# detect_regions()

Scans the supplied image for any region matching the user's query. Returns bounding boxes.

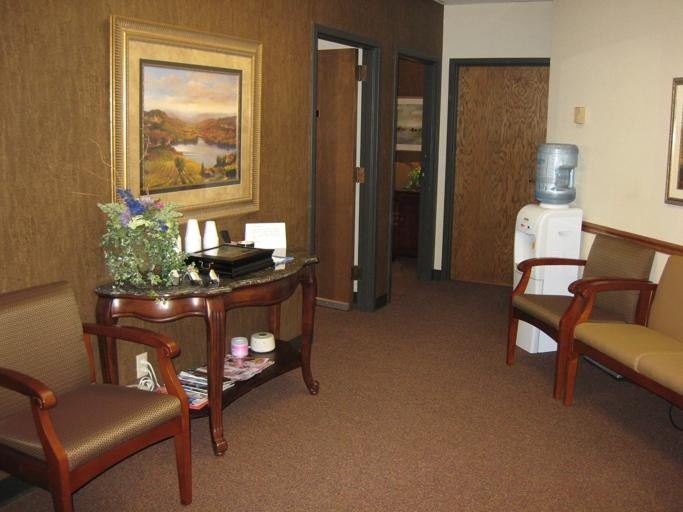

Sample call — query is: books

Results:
[151,352,275,411]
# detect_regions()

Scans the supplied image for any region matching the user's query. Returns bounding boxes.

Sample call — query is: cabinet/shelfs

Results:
[394,190,418,258]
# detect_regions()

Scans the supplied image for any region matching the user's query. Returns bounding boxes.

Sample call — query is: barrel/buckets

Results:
[533,143,579,204]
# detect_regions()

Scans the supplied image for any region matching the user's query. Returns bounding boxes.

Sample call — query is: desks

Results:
[91,253,321,453]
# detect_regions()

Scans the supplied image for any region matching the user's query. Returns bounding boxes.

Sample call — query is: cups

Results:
[183,218,221,251]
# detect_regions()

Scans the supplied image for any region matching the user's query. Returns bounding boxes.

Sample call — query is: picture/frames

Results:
[396,96,422,151]
[109,14,263,229]
[664,78,682,204]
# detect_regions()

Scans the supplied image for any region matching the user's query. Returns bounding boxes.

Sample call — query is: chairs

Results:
[506,220,683,412]
[2,280,191,512]
